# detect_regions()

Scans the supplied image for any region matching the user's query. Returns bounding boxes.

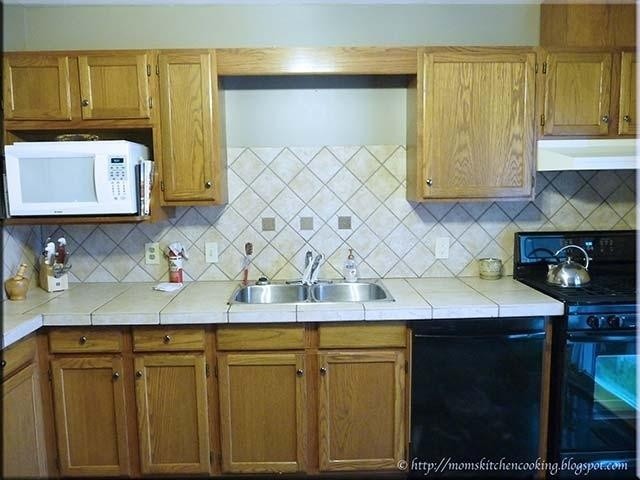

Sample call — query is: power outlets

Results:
[435,236,449,261]
[144,243,160,265]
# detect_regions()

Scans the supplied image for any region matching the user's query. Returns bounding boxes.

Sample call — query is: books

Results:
[145,159,154,217]
[133,162,141,217]
[140,160,145,217]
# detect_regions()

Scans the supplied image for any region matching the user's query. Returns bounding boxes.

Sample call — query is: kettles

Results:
[546,245,594,289]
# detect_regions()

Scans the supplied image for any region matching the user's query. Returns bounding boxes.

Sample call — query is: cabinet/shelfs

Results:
[406,54,534,204]
[132,329,214,479]
[48,329,126,477]
[0,333,49,479]
[160,50,229,208]
[314,324,405,472]
[1,52,153,121]
[215,327,310,476]
[536,4,638,139]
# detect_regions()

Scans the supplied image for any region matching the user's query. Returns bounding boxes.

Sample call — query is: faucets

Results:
[304,251,322,282]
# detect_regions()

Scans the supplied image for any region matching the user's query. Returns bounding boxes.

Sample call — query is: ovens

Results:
[551,330,640,478]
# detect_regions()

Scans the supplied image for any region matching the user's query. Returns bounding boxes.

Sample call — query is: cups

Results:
[478,257,501,281]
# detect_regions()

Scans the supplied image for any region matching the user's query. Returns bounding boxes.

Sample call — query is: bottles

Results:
[168,243,183,283]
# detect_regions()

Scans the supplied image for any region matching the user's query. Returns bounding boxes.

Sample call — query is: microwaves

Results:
[4,139,149,218]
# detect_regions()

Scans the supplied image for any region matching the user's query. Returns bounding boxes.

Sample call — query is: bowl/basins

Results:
[5,277,29,301]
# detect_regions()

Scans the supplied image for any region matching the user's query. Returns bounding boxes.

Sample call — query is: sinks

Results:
[310,278,396,303]
[227,280,310,305]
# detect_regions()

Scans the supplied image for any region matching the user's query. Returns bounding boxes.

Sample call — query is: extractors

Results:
[537,140,639,173]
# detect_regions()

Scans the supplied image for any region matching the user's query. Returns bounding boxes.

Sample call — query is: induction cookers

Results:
[519,273,637,331]
